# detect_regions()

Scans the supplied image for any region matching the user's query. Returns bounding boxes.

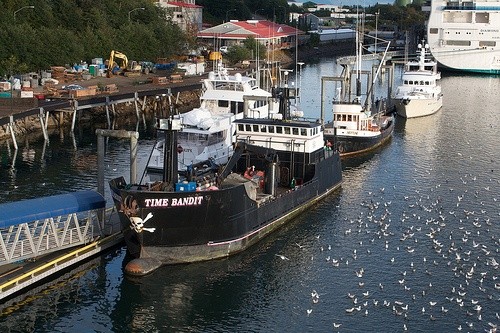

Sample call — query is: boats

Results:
[108,85,341,277]
[391,40,444,119]
[423,0,500,75]
[143,21,309,184]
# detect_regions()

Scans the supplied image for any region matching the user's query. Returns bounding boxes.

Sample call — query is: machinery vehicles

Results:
[105,49,143,80]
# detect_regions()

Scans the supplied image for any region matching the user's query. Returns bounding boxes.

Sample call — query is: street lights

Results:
[128,8,145,23]
[226,9,236,21]
[13,6,34,22]
[279,6,286,23]
[255,9,264,20]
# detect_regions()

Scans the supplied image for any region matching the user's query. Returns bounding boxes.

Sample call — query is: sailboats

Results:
[319,2,408,160]
